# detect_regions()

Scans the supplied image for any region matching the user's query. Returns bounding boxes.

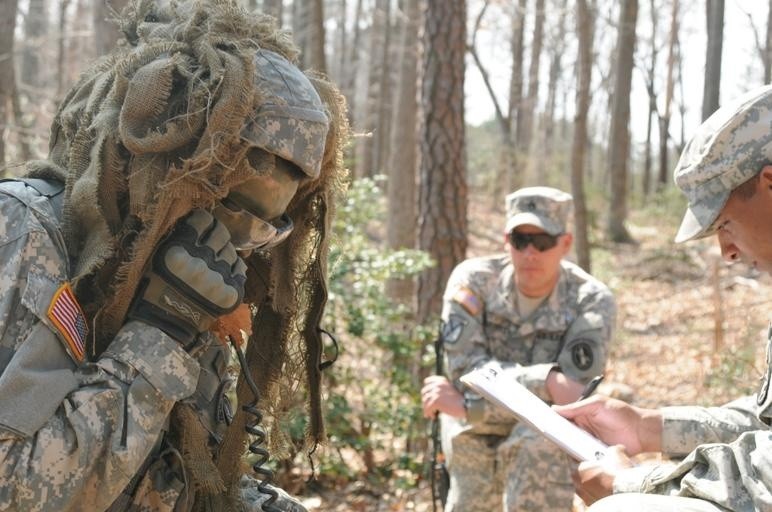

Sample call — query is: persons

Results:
[542,84,772,511]
[418,184,615,512]
[0,0,376,511]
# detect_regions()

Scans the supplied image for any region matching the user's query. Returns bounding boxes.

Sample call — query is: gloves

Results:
[131,209,245,348]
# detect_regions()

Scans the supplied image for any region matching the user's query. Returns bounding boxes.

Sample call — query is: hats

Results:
[673,84,772,245]
[503,186,573,236]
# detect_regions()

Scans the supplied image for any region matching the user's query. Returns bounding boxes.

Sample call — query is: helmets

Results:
[233,45,327,181]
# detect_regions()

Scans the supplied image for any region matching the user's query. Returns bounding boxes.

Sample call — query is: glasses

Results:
[507,231,562,252]
[205,198,295,252]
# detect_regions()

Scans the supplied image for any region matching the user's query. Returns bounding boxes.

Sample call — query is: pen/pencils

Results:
[579,375,604,400]
